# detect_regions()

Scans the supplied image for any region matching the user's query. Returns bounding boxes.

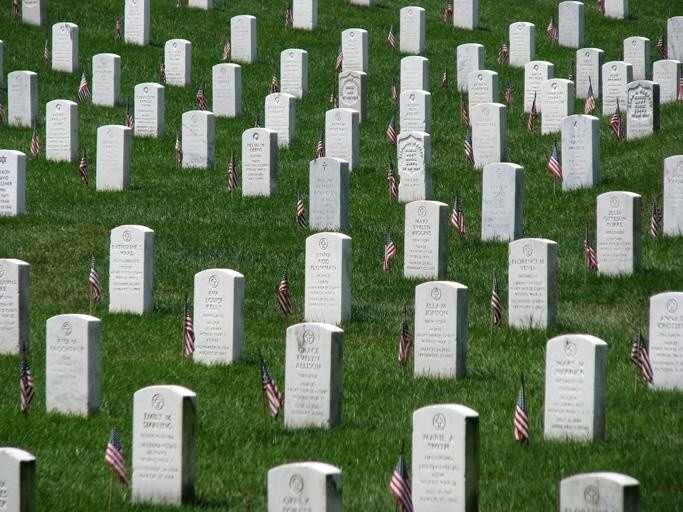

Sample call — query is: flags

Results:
[104,425,130,486]
[87,254,102,302]
[18,352,36,414]
[182,304,197,358]
[395,317,414,365]
[490,277,503,328]
[387,453,414,511]
[258,358,283,420]
[275,268,294,317]
[13,1,683,279]
[628,324,655,388]
[512,383,531,444]
